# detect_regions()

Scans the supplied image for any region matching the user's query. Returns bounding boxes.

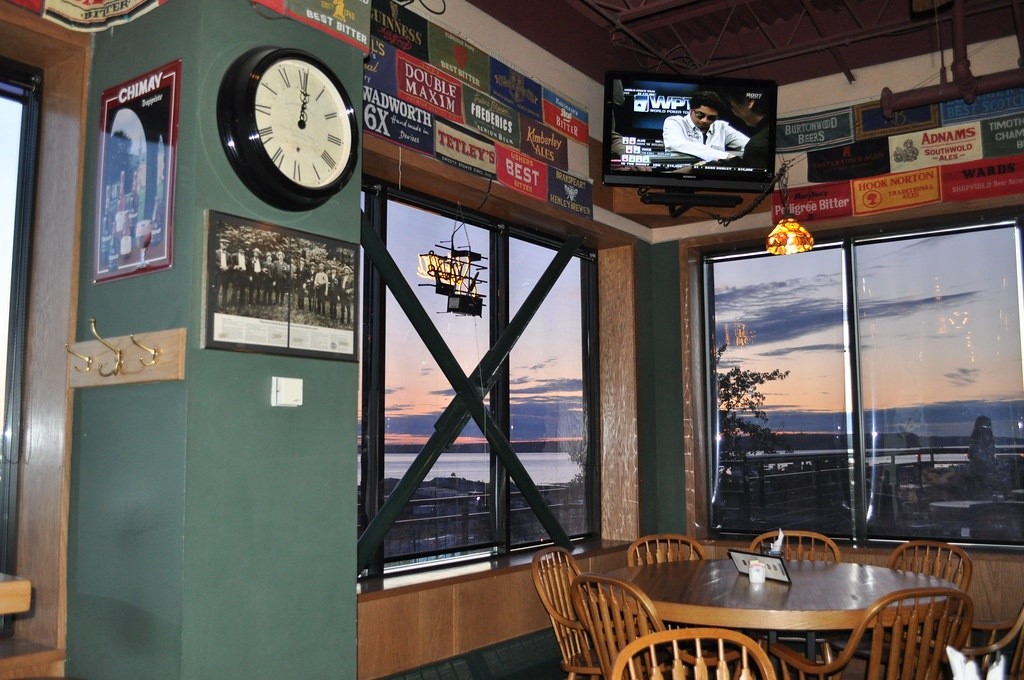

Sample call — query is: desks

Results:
[927,499,1024,537]
[581,558,964,680]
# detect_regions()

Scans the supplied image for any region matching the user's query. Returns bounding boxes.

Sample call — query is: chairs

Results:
[872,484,1024,540]
[532,534,1024,680]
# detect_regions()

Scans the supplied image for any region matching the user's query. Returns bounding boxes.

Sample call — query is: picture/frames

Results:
[202,206,366,365]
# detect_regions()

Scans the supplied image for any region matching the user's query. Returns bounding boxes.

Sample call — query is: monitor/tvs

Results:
[602,71,778,194]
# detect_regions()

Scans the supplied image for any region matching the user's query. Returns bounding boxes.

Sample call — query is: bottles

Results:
[115,171,129,243]
[128,184,138,247]
[150,196,163,246]
[100,184,112,268]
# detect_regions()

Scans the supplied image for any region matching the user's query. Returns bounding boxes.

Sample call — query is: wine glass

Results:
[136,218,152,263]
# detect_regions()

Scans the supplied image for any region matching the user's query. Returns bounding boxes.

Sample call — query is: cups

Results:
[120,235,133,265]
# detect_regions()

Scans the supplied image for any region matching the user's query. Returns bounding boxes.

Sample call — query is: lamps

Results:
[765,172,815,254]
[413,200,489,318]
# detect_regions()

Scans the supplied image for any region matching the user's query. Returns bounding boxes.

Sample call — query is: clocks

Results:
[216,46,360,211]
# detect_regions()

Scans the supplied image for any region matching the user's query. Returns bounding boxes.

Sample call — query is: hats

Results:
[253,248,262,256]
[330,266,339,271]
[309,262,316,266]
[219,239,230,246]
[343,266,351,273]
[319,264,325,267]
[277,252,285,259]
[300,258,305,261]
[238,245,246,251]
[266,252,273,257]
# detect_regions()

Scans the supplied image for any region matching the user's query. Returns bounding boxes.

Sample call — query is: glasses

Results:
[694,107,720,120]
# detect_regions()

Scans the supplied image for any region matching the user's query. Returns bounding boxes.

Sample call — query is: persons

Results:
[663,87,771,174]
[215,238,353,324]
